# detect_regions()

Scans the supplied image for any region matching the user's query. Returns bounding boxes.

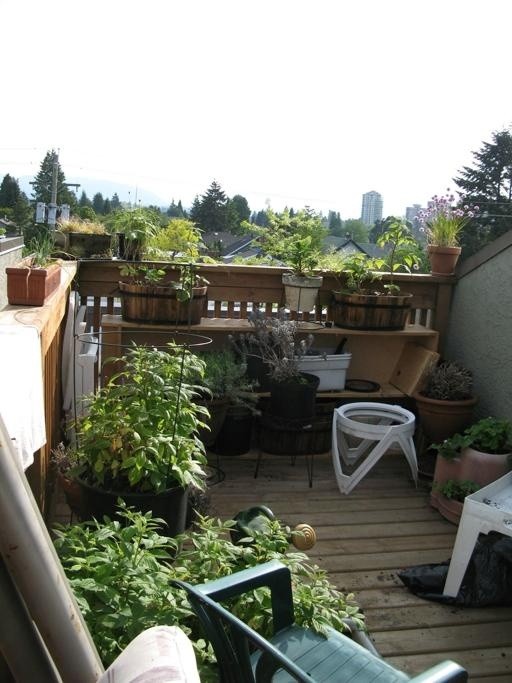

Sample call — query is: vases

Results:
[270,372,320,420]
[427,244,462,276]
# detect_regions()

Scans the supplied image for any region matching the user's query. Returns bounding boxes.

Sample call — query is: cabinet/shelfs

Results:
[98,314,440,460]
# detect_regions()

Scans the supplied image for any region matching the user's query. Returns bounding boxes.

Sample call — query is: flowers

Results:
[414,187,480,247]
[228,307,315,383]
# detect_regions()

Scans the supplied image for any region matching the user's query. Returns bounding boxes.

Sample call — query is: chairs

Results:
[168,559,470,681]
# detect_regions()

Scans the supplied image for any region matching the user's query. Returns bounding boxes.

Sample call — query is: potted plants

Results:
[240,205,423,331]
[5,207,208,325]
[415,358,512,527]
